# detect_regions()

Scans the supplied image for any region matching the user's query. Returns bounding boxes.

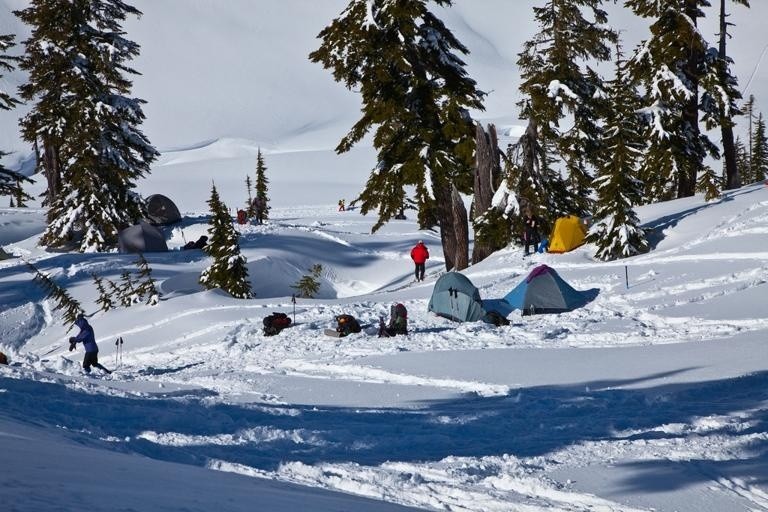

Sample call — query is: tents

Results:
[142,193,182,225]
[547,215,587,253]
[503,265,585,313]
[427,272,484,323]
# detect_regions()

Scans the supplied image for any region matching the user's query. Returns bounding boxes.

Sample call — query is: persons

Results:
[68,316,111,375]
[253,193,265,223]
[339,199,346,211]
[411,240,430,283]
[522,207,539,255]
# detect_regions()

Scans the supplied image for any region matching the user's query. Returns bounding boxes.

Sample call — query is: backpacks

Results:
[336,314,362,337]
[377,302,408,338]
[263,312,292,336]
